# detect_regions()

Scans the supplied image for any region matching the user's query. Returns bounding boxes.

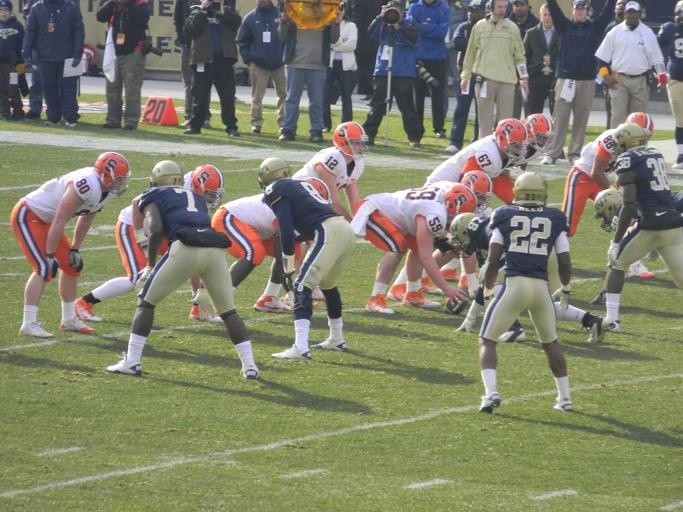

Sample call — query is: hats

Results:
[0,0,12,7]
[624,1,640,11]
[573,0,589,9]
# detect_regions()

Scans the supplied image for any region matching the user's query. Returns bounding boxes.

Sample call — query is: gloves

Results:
[189,289,216,321]
[283,274,297,292]
[137,267,151,283]
[589,292,603,305]
[69,251,83,270]
[72,58,80,67]
[47,253,59,279]
[456,273,495,333]
[608,240,619,266]
[552,284,571,311]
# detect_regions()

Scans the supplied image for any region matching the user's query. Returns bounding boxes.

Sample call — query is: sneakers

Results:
[630,260,656,278]
[180,117,329,141]
[0,110,79,127]
[241,363,259,378]
[671,162,683,168]
[410,131,460,153]
[254,289,346,360]
[497,328,527,341]
[106,351,141,375]
[542,155,555,163]
[479,392,501,410]
[190,304,223,321]
[20,297,103,337]
[553,398,573,410]
[582,314,621,343]
[366,270,468,314]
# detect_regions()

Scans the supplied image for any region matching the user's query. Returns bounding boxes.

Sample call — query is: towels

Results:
[102,26,117,83]
[560,78,576,102]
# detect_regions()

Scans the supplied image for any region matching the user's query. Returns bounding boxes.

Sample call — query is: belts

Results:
[618,72,647,77]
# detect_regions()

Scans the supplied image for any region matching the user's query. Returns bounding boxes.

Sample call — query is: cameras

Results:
[143,39,163,57]
[210,1,221,12]
[416,61,440,89]
[386,8,401,24]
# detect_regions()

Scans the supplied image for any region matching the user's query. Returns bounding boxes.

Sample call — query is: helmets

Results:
[613,112,654,152]
[96,152,224,202]
[257,122,368,200]
[674,0,683,21]
[594,188,623,232]
[444,113,555,258]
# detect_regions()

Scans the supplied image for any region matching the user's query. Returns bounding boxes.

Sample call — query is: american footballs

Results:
[445,286,469,315]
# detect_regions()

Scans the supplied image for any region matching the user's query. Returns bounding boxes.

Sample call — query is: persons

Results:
[598,123,683,333]
[386,170,493,308]
[75,164,223,323]
[0,1,86,129]
[351,181,477,313]
[490,113,551,206]
[94,2,154,130]
[560,112,657,278]
[211,176,331,323]
[423,118,529,288]
[174,0,682,166]
[257,157,355,359]
[283,121,369,309]
[479,172,575,412]
[11,152,132,338]
[448,213,603,342]
[106,160,259,379]
